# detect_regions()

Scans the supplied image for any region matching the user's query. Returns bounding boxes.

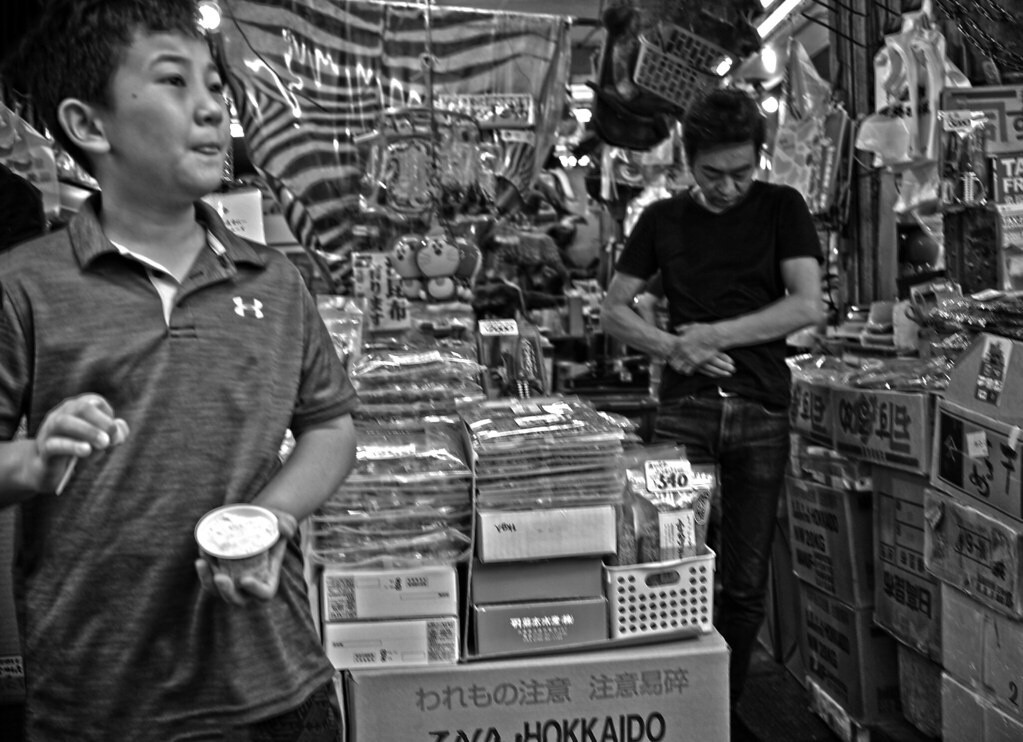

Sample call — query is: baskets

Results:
[601,544,716,640]
[633,22,738,109]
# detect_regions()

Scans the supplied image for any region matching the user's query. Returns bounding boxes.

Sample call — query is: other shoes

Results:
[730,710,764,742]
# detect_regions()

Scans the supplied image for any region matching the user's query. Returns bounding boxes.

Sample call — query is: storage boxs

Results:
[601,543,717,636]
[632,20,741,114]
[341,619,733,741]
[783,324,1023,742]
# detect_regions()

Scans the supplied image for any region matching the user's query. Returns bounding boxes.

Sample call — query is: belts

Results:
[699,384,750,400]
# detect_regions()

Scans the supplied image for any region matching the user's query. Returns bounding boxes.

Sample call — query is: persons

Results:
[521,228,569,276]
[599,88,829,725]
[0,0,359,742]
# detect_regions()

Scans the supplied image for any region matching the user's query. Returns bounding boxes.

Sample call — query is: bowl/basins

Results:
[195,503,280,589]
